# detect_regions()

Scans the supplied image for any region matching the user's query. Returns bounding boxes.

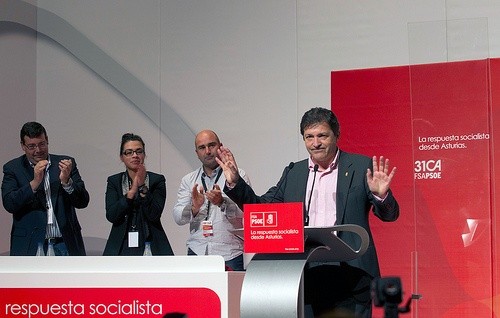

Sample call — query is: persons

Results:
[172,130,250,271]
[215,107,399,318]
[101,133,176,255]
[1,121,90,256]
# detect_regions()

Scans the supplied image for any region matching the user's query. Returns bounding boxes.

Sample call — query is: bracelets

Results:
[61,178,72,187]
[138,183,147,194]
[218,199,227,211]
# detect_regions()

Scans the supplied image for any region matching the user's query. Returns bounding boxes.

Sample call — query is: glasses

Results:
[24,142,47,150]
[122,148,144,156]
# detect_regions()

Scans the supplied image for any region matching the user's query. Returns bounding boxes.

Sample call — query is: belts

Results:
[45,237,64,245]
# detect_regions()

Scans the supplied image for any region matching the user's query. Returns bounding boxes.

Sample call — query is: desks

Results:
[0,254,248,318]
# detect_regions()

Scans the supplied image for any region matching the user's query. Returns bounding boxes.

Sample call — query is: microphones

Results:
[305,164,319,226]
[270,162,294,203]
